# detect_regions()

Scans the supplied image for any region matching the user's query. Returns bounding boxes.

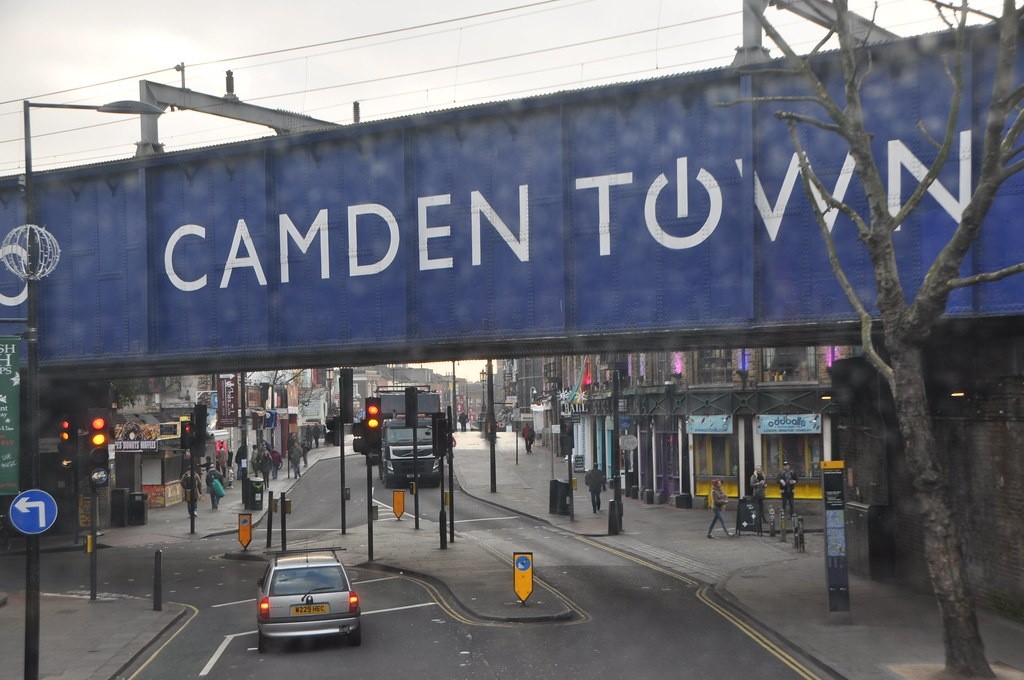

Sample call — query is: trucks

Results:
[374,386,442,488]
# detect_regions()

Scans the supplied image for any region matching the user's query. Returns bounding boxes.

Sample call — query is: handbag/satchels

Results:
[212,479,226,498]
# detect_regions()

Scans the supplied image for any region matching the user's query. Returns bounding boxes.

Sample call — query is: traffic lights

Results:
[57,414,75,469]
[560,421,574,449]
[365,397,382,465]
[86,408,108,486]
[181,421,193,449]
[193,404,209,457]
[326,414,339,446]
[353,420,365,455]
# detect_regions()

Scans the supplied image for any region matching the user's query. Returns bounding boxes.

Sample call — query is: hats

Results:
[711,480,720,485]
[783,459,790,465]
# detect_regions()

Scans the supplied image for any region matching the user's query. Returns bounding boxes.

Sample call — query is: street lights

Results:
[480,370,486,411]
[530,387,537,403]
[23,100,165,680]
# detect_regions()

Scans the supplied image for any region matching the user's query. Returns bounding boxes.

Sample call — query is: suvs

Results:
[256,547,361,652]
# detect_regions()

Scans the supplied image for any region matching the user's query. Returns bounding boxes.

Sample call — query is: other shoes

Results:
[194,511,198,517]
[728,532,735,538]
[706,533,715,538]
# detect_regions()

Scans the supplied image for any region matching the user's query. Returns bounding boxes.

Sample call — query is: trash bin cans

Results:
[246,477,264,510]
[127,491,148,526]
[548,478,572,516]
[111,487,130,527]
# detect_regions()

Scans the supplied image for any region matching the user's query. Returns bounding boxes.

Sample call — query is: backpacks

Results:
[183,472,197,489]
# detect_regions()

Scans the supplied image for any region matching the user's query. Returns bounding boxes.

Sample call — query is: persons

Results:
[250,423,321,490]
[751,464,769,524]
[180,465,202,519]
[584,463,606,513]
[458,409,469,432]
[521,423,534,454]
[776,461,798,518]
[207,461,221,510]
[706,479,736,539]
[215,439,245,488]
